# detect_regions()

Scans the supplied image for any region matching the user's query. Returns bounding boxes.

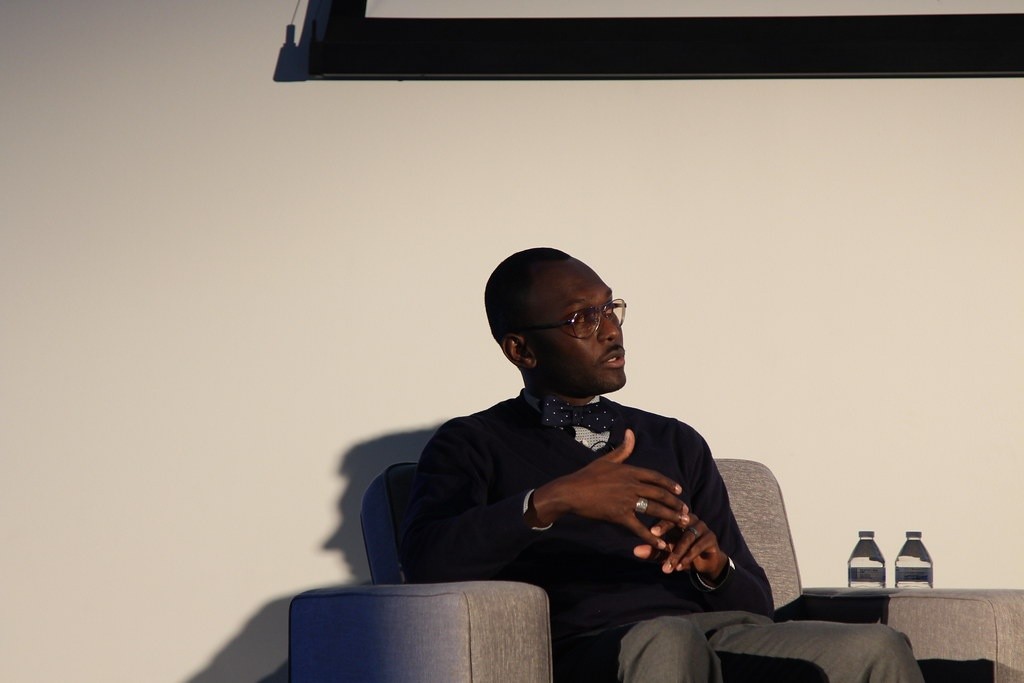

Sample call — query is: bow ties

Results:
[522,390,617,433]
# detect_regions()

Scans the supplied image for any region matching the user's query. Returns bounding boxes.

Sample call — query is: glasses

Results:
[517,299,626,340]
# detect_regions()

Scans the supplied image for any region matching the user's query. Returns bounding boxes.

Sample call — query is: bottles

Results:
[848,530,885,589]
[895,531,934,589]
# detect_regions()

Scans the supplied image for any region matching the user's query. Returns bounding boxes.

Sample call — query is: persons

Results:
[399,247,925,683]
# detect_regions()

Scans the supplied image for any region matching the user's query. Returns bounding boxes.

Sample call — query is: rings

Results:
[685,526,698,540]
[635,498,649,513]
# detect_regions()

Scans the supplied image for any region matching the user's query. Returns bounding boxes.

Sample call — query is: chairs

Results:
[288,458,1024,683]
